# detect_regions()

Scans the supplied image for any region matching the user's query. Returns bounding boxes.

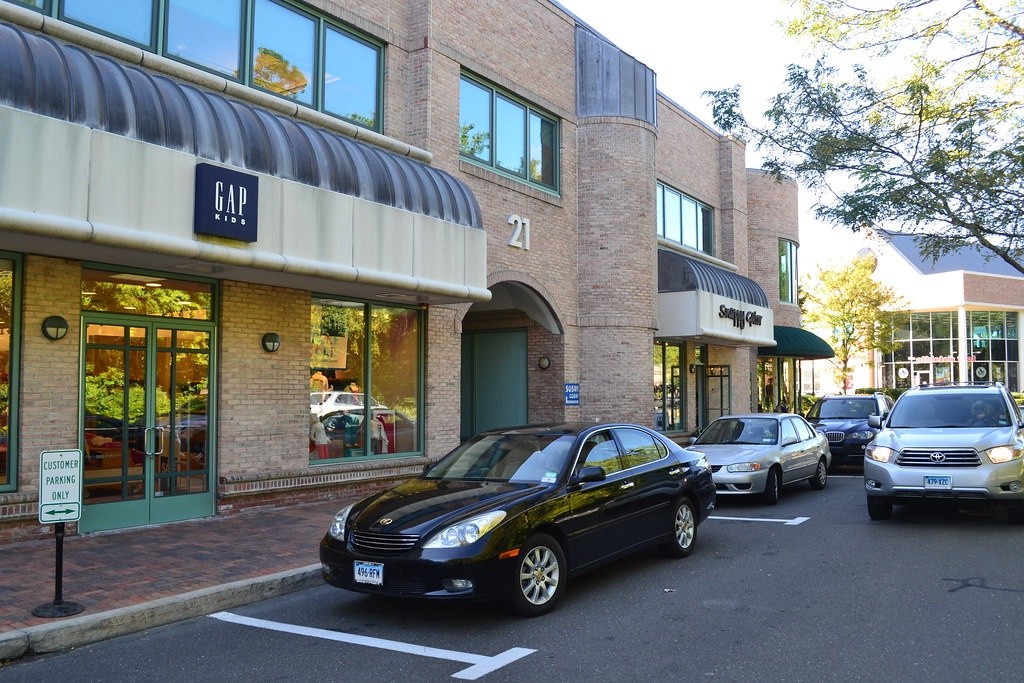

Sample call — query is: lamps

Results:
[262,333,280,352]
[538,356,550,369]
[42,315,69,341]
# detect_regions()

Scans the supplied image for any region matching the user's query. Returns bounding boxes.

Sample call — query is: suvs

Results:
[805,392,895,471]
[144,395,208,468]
[863,383,1024,520]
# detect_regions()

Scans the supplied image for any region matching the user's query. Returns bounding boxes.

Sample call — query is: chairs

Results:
[910,404,945,427]
[728,422,776,443]
[484,438,602,486]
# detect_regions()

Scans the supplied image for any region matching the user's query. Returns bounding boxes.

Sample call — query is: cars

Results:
[655,393,682,407]
[310,392,366,420]
[320,409,415,451]
[685,413,831,505]
[658,408,670,425]
[936,380,944,382]
[84,413,146,448]
[354,394,389,409]
[322,424,717,616]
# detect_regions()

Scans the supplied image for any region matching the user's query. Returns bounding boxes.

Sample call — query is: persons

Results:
[371,419,389,454]
[971,401,998,426]
[310,372,328,405]
[765,378,773,411]
[310,418,331,459]
[341,383,363,447]
